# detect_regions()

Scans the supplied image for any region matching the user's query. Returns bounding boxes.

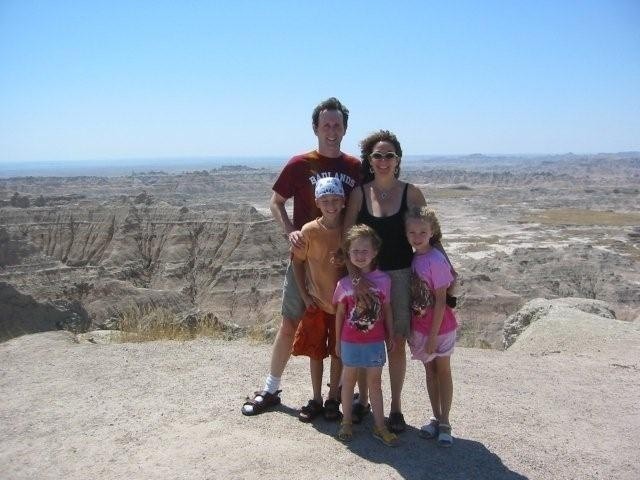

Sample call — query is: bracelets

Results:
[351,273,362,288]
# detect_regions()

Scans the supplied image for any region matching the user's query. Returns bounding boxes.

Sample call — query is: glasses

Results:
[370,151,399,162]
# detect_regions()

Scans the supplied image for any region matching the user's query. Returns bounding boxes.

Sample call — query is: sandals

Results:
[241,390,283,416]
[299,387,452,448]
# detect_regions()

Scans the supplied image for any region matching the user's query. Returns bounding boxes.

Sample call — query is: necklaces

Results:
[370,181,394,202]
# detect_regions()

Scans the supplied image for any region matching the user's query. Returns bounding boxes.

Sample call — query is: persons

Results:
[339,128,458,431]
[242,96,363,417]
[406,201,456,446]
[290,177,347,423]
[334,221,399,447]
